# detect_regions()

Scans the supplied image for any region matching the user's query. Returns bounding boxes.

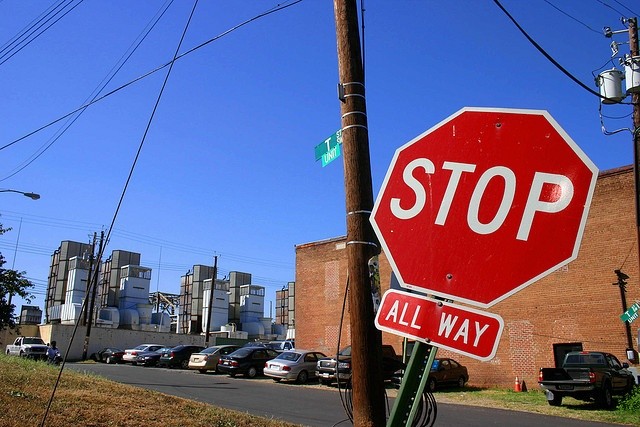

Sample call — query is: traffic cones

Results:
[515,374,520,391]
[522,380,527,390]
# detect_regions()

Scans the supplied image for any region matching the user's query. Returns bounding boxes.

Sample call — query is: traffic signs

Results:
[620,303,640,322]
[313,129,342,167]
[375,288,503,361]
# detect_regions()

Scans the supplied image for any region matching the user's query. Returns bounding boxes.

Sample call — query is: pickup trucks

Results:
[315,344,411,386]
[5,336,50,359]
[538,351,635,407]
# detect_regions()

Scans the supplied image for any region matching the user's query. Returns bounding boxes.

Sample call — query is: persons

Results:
[48,340,59,363]
[44,342,50,361]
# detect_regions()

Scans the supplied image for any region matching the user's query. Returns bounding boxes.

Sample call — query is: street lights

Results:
[0,187,45,204]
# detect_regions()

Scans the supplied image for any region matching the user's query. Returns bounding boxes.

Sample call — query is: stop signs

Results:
[368,106,599,308]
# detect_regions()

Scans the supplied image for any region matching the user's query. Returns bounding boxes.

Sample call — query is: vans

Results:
[269,339,294,349]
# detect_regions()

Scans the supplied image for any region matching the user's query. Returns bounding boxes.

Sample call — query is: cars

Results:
[188,344,239,372]
[263,350,327,383]
[91,347,124,363]
[217,346,279,378]
[137,347,169,366]
[122,343,165,364]
[160,344,206,368]
[391,357,468,391]
